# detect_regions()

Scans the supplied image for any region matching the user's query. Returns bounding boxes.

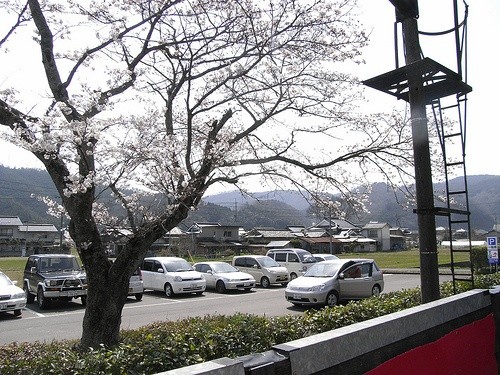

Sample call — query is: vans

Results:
[233,255,290,288]
[266,248,340,281]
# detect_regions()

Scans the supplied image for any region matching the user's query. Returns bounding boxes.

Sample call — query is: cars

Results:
[141,256,206,298]
[193,262,256,294]
[107,257,144,300]
[284,259,385,309]
[0,272,27,316]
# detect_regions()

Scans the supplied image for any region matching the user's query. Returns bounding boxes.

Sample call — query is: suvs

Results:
[22,254,87,309]
